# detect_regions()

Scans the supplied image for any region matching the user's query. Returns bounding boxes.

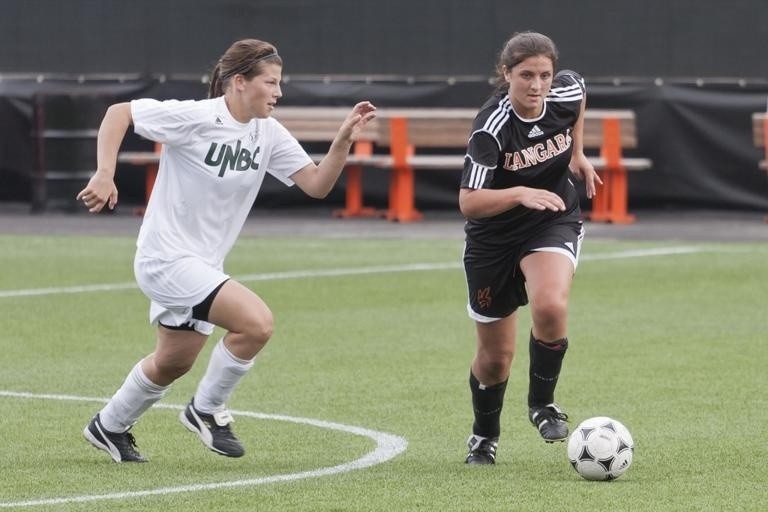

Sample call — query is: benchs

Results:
[117,98,654,226]
[749,111,766,174]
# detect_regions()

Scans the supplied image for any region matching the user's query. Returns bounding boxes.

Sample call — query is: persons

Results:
[78,35,380,465]
[456,28,612,469]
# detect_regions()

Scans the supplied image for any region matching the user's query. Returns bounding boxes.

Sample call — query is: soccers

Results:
[568,418,635,479]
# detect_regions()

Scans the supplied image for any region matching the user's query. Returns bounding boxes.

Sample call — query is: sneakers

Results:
[526,402,573,445]
[78,409,149,468]
[175,391,247,463]
[462,431,501,467]
